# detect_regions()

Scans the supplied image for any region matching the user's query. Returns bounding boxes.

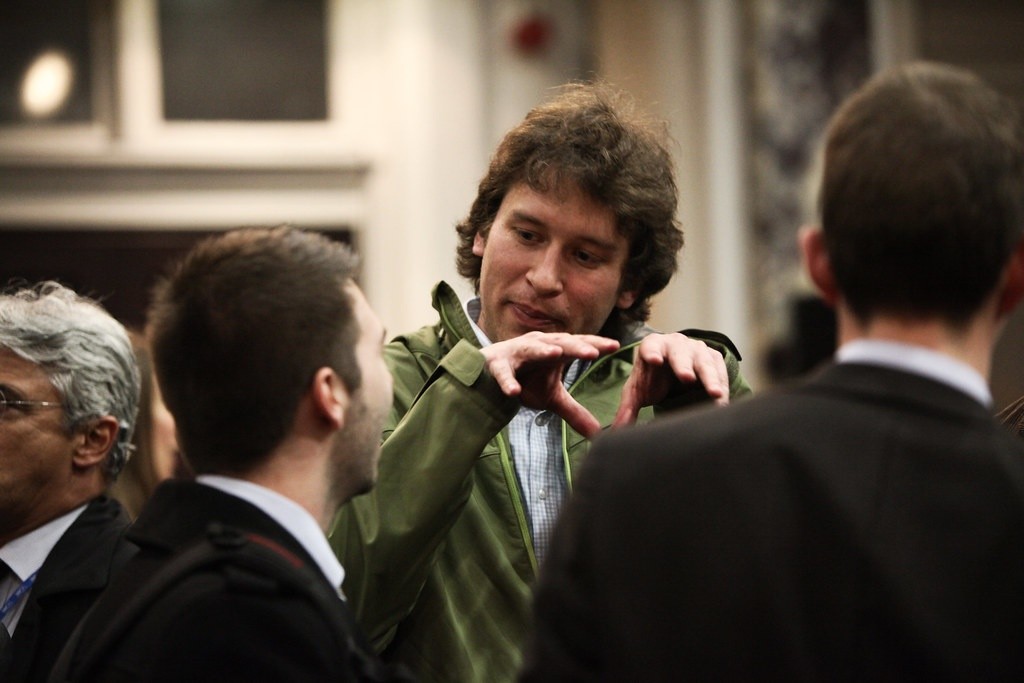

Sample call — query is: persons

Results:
[2,289,141,683]
[322,93,752,683]
[527,56,1024,683]
[50,227,386,683]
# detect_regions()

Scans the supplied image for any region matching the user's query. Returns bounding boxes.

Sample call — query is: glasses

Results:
[0,389,67,427]
